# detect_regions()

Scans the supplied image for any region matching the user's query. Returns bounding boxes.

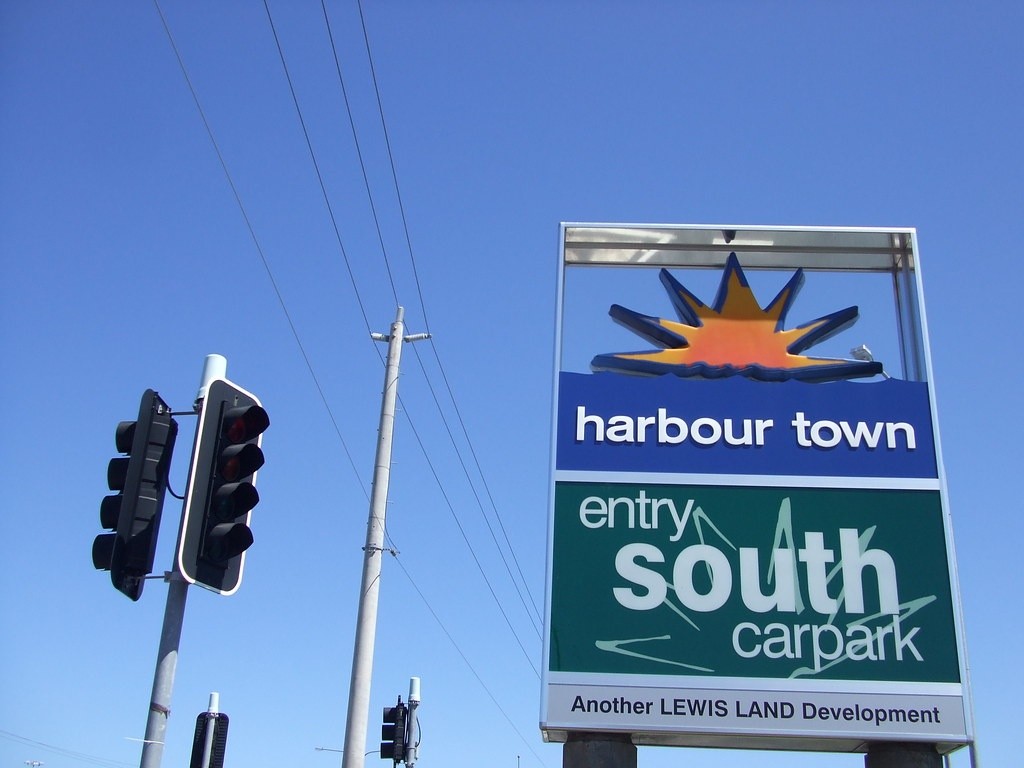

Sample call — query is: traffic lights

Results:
[177,374,270,596]
[380,702,408,759]
[92,385,179,601]
